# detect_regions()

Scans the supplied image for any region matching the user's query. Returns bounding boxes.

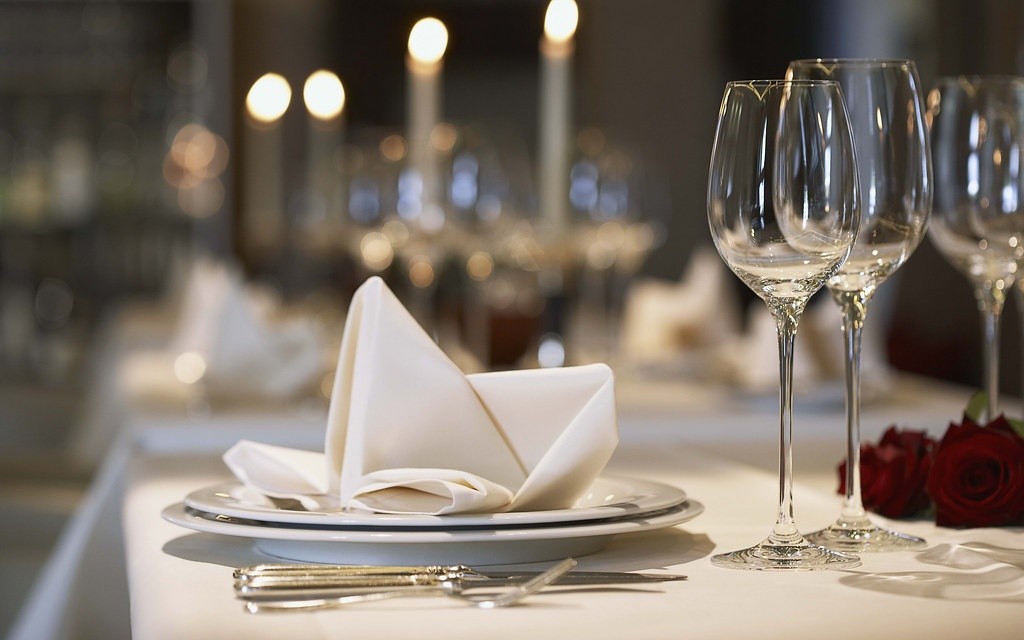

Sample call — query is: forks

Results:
[228,556,577,609]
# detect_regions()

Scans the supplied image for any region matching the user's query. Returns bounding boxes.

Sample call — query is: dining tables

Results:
[122,441,1024,640]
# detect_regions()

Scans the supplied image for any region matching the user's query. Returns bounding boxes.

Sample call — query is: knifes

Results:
[236,558,689,585]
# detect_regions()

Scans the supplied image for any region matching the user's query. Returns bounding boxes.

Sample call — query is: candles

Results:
[538,0,580,226]
[405,17,448,193]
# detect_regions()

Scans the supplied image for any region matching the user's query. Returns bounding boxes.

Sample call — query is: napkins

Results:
[220,278,619,517]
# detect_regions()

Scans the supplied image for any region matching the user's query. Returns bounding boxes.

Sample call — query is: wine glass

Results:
[928,76,1024,419]
[706,80,862,572]
[773,57,934,553]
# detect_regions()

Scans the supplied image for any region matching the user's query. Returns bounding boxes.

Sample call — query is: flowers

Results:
[837,385,1024,529]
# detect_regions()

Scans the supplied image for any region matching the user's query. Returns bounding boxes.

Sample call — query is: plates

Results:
[161,473,705,566]
